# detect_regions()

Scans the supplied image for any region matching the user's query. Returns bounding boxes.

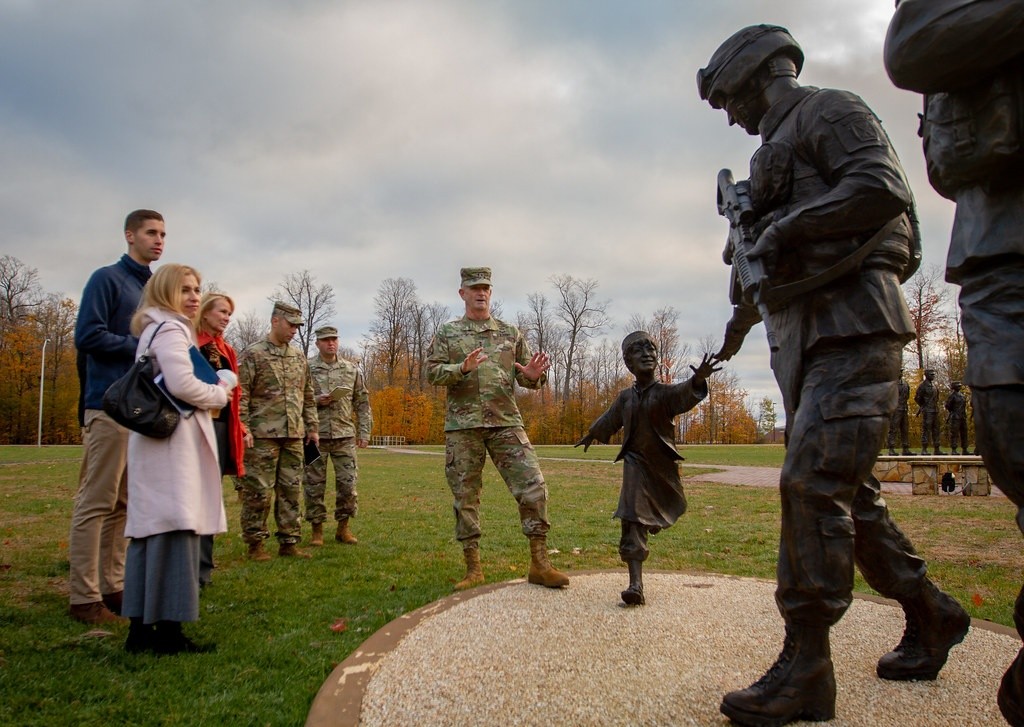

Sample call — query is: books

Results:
[304,439,320,466]
[153,345,228,419]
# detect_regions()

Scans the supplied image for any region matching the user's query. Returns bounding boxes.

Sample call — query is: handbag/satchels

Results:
[102,322,180,438]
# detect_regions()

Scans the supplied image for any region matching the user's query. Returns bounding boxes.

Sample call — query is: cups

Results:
[216,369,238,390]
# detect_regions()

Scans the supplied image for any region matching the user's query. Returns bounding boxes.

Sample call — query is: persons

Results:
[888,366,917,456]
[304,326,373,544]
[194,294,246,587]
[695,26,970,726]
[66,210,167,620]
[237,301,320,560]
[885,0,1024,727]
[575,332,724,603]
[946,380,973,455]
[121,263,233,653]
[427,267,576,588]
[915,369,946,455]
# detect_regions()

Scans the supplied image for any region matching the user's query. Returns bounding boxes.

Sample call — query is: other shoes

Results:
[248,548,272,560]
[102,592,123,614]
[68,601,119,625]
[279,544,312,558]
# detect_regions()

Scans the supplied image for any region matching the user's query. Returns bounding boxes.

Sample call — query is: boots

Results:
[335,518,358,544]
[309,522,324,545]
[125,617,152,654]
[529,538,570,587]
[153,622,216,656]
[454,547,485,590]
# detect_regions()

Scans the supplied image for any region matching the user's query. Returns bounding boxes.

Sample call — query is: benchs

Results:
[909,460,992,498]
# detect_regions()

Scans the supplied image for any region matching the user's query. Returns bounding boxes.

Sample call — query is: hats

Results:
[316,326,339,339]
[460,267,493,287]
[274,300,305,327]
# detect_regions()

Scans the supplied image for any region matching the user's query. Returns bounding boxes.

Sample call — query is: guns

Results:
[717,168,781,353]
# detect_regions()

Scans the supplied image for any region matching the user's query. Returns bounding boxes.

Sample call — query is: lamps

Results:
[941,472,957,493]
